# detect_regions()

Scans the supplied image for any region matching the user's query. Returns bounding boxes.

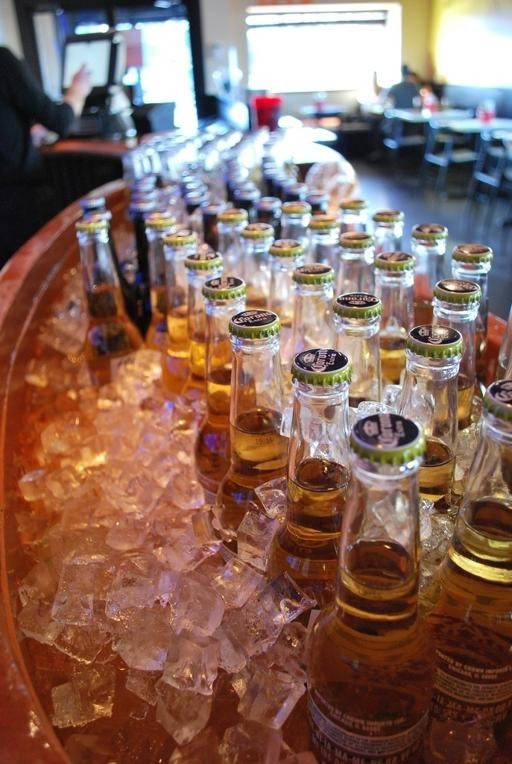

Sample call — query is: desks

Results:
[301,95,512,254]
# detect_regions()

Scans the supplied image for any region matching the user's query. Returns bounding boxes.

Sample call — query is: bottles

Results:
[71,124,512,764]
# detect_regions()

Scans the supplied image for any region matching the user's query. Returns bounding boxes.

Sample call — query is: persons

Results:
[383,62,422,107]
[0,43,97,260]
[409,69,424,91]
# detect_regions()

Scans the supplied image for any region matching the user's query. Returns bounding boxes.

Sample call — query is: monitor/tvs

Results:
[61,31,120,117]
